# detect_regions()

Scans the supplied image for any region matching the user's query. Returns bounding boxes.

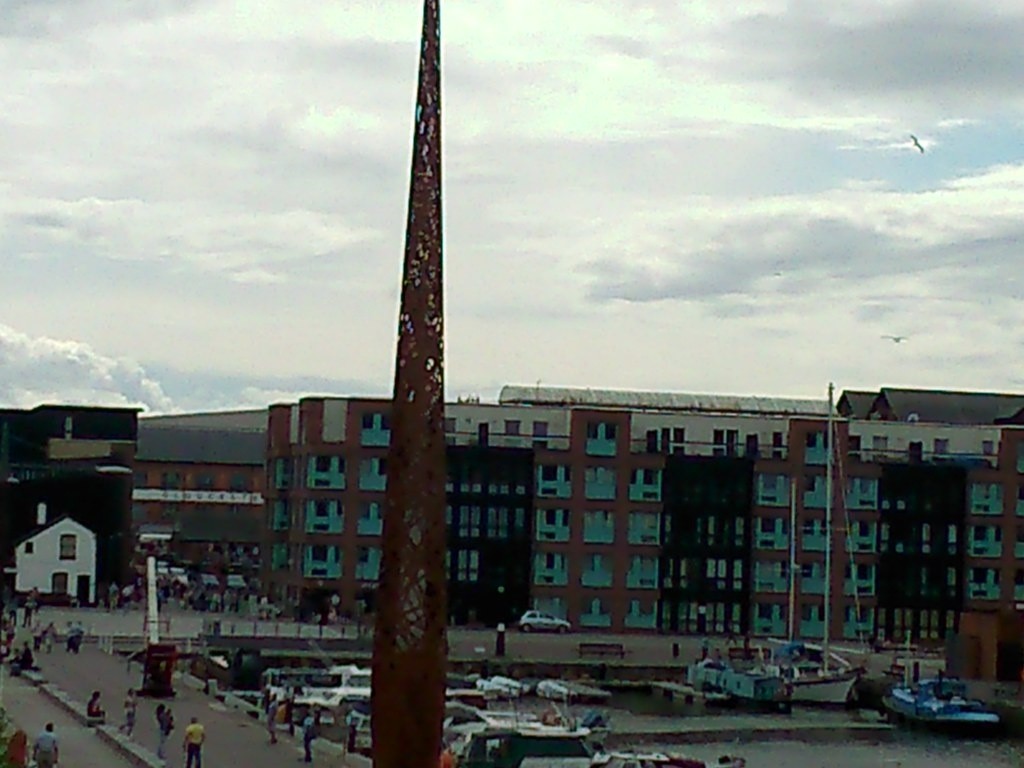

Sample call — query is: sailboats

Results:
[748,382,871,706]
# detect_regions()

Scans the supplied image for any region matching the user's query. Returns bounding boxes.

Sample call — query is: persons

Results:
[993,679,1020,699]
[88,692,104,719]
[0,585,85,677]
[157,703,174,759]
[4,730,30,768]
[183,717,206,768]
[107,574,268,621]
[701,634,709,659]
[260,683,316,762]
[441,733,468,768]
[33,722,58,768]
[725,623,737,646]
[119,689,139,737]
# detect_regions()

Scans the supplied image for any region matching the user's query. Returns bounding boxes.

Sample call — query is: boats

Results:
[879,629,1003,736]
[686,650,787,707]
[535,678,612,707]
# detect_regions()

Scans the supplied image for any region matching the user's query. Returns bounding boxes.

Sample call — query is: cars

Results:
[157,561,170,573]
[201,575,220,587]
[227,575,246,590]
[519,609,573,634]
[261,658,757,768]
[170,568,185,577]
